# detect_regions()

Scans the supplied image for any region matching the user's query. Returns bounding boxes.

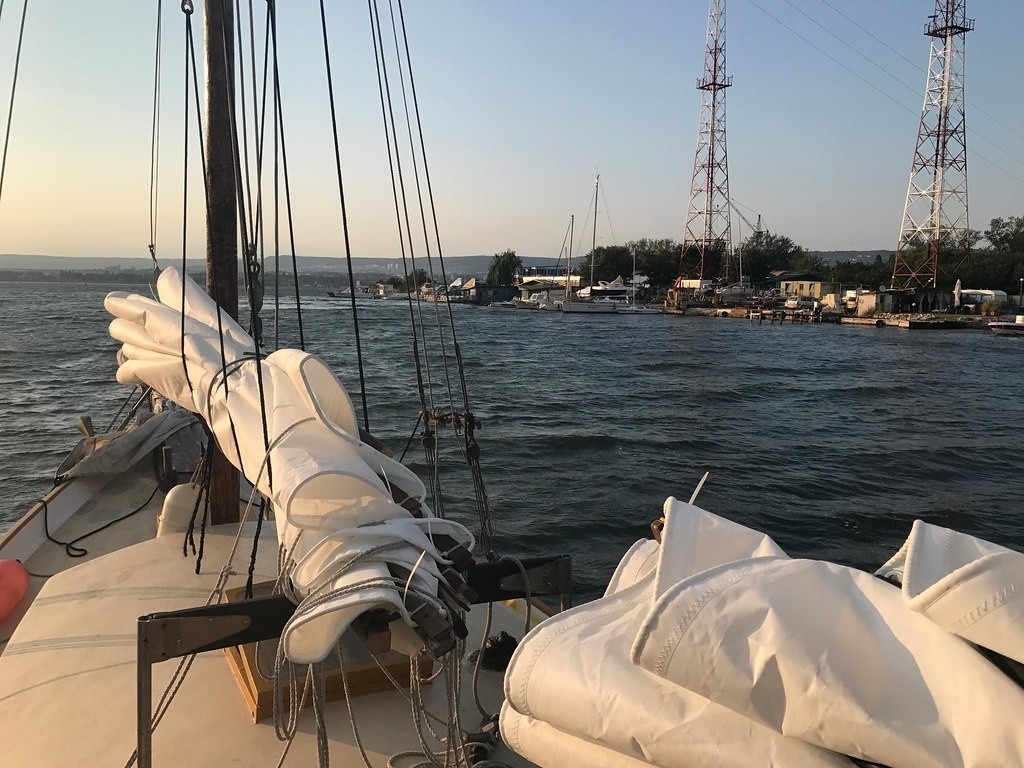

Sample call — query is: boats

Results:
[0,2,1022,768]
